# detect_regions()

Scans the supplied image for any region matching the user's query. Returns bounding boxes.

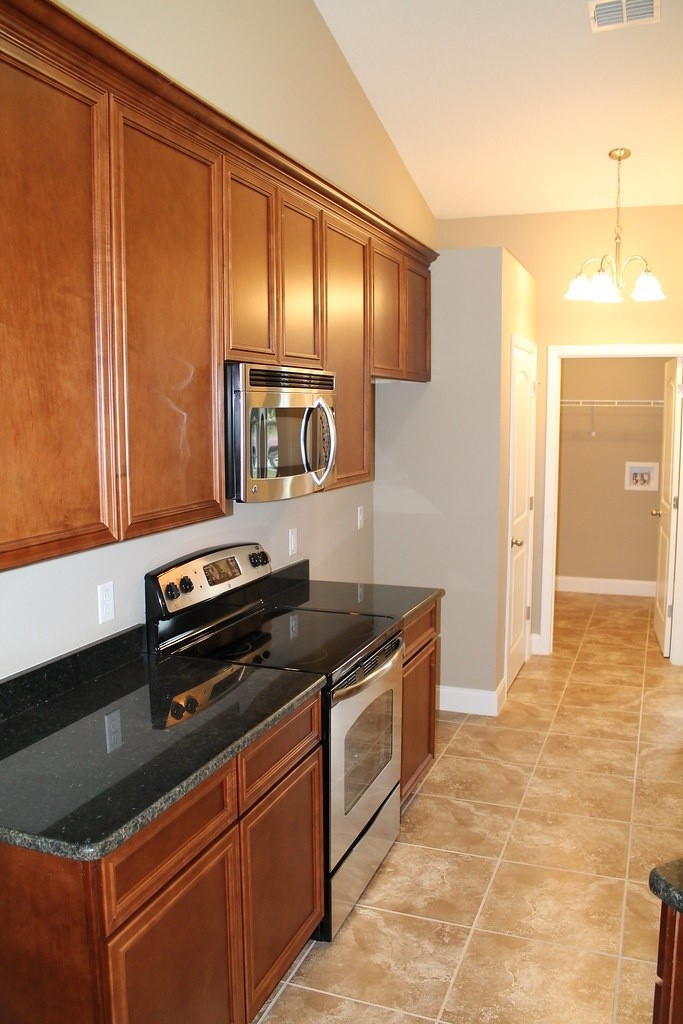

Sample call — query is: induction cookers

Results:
[144,539,405,685]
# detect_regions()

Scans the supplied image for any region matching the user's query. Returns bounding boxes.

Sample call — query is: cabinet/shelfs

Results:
[322,209,375,491]
[0,30,234,571]
[0,691,324,1024]
[400,599,437,806]
[370,237,432,382]
[222,155,324,369]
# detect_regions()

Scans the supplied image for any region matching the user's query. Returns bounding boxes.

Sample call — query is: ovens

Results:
[322,631,401,942]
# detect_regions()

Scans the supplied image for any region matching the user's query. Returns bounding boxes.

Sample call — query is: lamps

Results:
[564,148,665,303]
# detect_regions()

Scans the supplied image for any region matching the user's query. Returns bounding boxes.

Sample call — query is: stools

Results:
[648,857,683,1024]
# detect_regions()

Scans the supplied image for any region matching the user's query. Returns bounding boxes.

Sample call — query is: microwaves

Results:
[226,363,338,502]
